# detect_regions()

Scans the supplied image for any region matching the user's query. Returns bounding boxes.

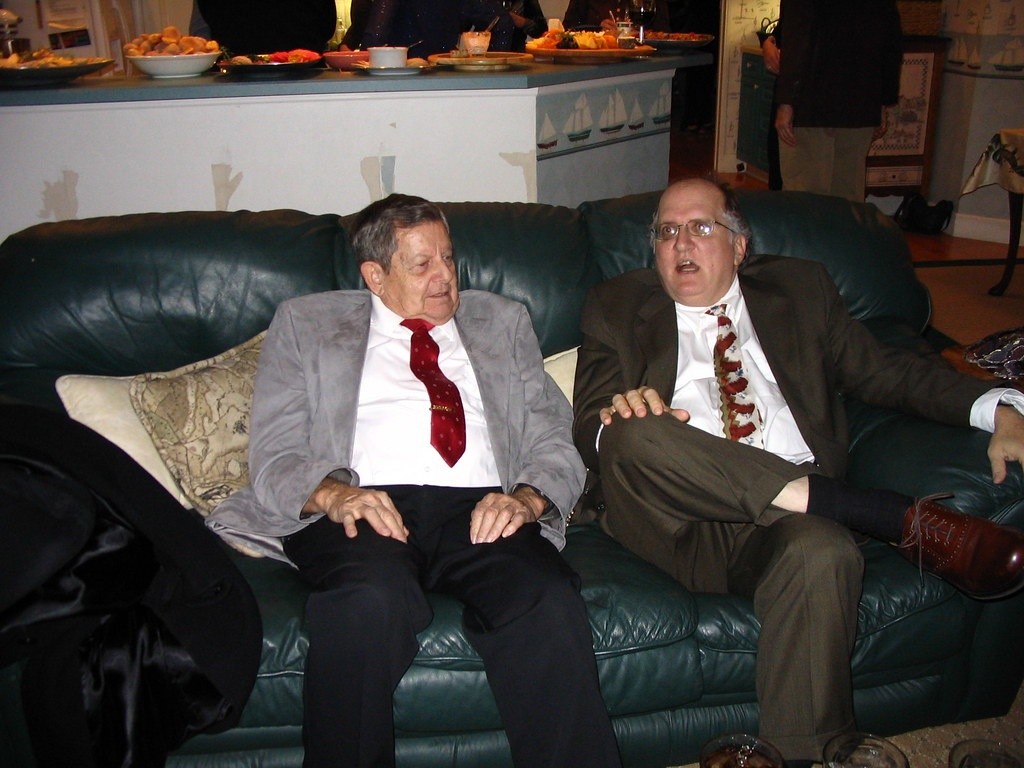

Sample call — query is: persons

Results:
[339,0,549,60]
[562,0,633,32]
[196,0,338,54]
[571,176,1024,768]
[203,194,628,768]
[775,0,904,202]
[763,23,780,76]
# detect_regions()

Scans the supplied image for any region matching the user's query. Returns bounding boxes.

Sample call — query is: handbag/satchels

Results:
[894,193,952,235]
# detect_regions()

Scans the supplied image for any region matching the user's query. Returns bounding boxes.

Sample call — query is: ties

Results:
[705,304,766,451]
[399,318,467,468]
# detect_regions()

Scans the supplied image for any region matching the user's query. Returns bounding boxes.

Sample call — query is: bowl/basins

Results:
[324,51,370,71]
[124,52,222,79]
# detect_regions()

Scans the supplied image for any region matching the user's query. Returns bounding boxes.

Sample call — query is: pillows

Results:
[54,326,580,560]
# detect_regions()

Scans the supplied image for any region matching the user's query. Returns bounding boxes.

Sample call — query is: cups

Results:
[618,37,635,48]
[367,47,408,67]
[462,32,491,58]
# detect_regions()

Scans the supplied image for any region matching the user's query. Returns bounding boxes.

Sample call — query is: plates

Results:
[0,59,115,90]
[354,64,441,75]
[642,35,714,57]
[215,54,332,81]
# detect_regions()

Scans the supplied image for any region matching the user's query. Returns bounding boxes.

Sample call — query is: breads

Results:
[123,26,219,57]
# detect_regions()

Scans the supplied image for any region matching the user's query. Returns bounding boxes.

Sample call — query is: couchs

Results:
[0,187,1024,768]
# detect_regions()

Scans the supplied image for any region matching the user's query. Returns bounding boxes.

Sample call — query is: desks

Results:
[958,130,1024,292]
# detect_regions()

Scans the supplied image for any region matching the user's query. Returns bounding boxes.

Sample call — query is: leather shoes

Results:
[889,490,1024,601]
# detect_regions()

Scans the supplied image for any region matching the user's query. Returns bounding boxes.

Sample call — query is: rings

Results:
[766,65,772,69]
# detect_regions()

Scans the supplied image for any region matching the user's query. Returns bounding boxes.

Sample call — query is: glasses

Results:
[653,217,735,238]
